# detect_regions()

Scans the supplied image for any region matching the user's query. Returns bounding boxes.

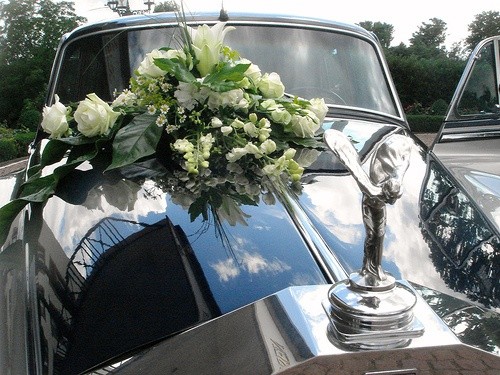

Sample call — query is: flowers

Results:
[0,19,328,250]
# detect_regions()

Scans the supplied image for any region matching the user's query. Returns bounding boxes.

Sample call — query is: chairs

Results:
[271,43,353,106]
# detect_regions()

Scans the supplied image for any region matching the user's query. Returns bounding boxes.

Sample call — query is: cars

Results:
[0,11,500,375]
